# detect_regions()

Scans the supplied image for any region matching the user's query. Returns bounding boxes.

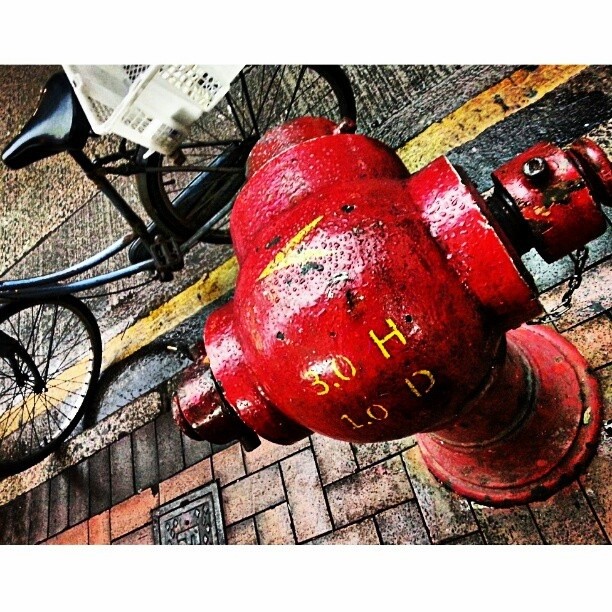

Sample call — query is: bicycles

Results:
[1,66,356,478]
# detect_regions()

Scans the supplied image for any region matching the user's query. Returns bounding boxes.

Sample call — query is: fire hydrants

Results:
[170,116,612,505]
